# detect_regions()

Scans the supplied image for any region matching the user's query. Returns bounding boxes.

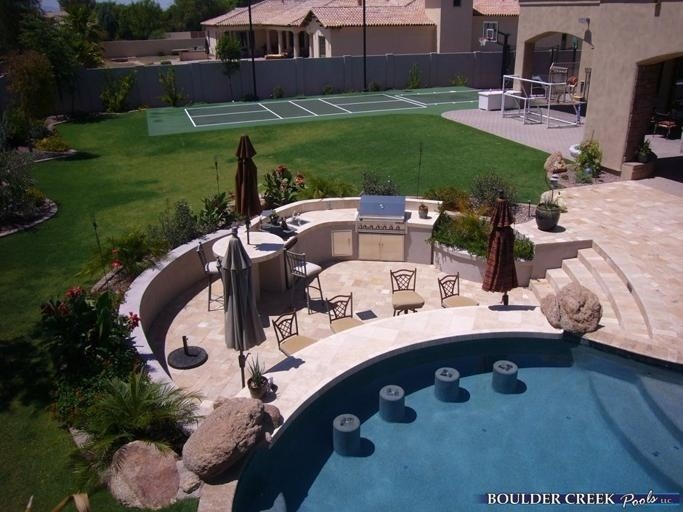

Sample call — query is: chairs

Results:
[654,110,678,138]
[390,268,479,317]
[272,293,365,357]
[285,250,323,314]
[196,242,224,312]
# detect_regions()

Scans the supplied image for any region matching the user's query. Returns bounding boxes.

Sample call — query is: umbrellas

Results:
[232,132,262,246]
[480,184,521,306]
[220,226,266,391]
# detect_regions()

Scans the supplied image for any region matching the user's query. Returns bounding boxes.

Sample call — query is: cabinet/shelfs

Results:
[331,230,352,257]
[358,233,405,262]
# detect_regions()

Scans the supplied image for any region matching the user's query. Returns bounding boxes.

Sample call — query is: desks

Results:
[212,232,284,302]
[478,91,522,111]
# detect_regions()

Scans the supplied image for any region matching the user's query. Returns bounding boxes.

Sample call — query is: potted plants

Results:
[247,351,268,400]
[535,191,566,231]
[638,139,651,163]
[576,129,602,178]
